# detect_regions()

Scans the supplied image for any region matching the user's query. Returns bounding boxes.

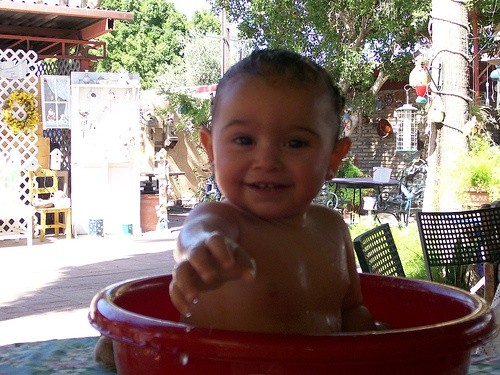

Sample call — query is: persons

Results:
[168,48,389,335]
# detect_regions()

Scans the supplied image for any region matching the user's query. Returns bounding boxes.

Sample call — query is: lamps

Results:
[393,85,417,154]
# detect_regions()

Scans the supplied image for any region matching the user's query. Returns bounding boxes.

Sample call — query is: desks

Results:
[330,177,399,223]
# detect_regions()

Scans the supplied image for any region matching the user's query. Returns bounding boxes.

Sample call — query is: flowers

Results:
[2,91,41,135]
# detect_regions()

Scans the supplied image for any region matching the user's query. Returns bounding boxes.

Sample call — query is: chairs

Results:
[414,204,500,281]
[354,222,406,277]
[28,169,71,241]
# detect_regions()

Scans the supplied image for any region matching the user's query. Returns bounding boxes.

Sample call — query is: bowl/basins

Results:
[88,273,495,375]
